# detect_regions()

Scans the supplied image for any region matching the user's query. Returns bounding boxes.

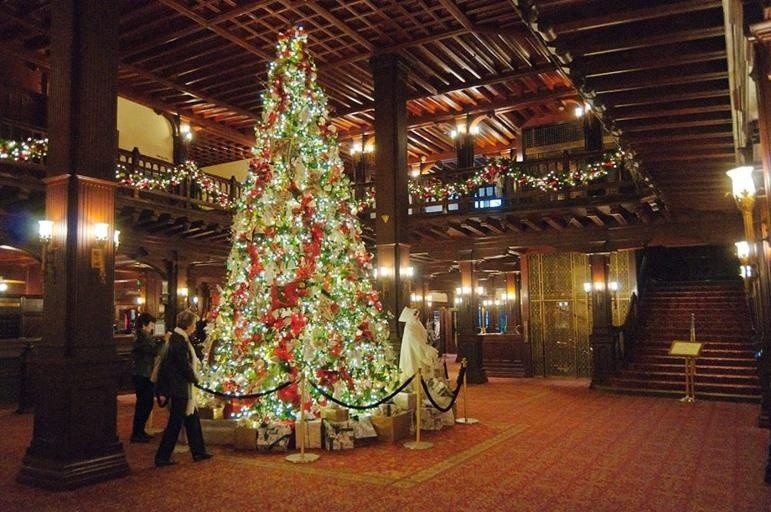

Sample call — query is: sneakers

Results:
[155,458,179,466]
[193,451,217,459]
[131,433,153,443]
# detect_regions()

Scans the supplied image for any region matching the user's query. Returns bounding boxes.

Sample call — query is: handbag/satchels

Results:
[156,361,171,395]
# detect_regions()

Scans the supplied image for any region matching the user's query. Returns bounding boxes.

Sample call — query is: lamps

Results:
[726,166,761,307]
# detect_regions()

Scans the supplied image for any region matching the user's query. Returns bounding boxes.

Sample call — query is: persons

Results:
[152,310,213,465]
[130,310,173,443]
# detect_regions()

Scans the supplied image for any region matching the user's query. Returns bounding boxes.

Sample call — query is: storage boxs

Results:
[196,390,455,450]
